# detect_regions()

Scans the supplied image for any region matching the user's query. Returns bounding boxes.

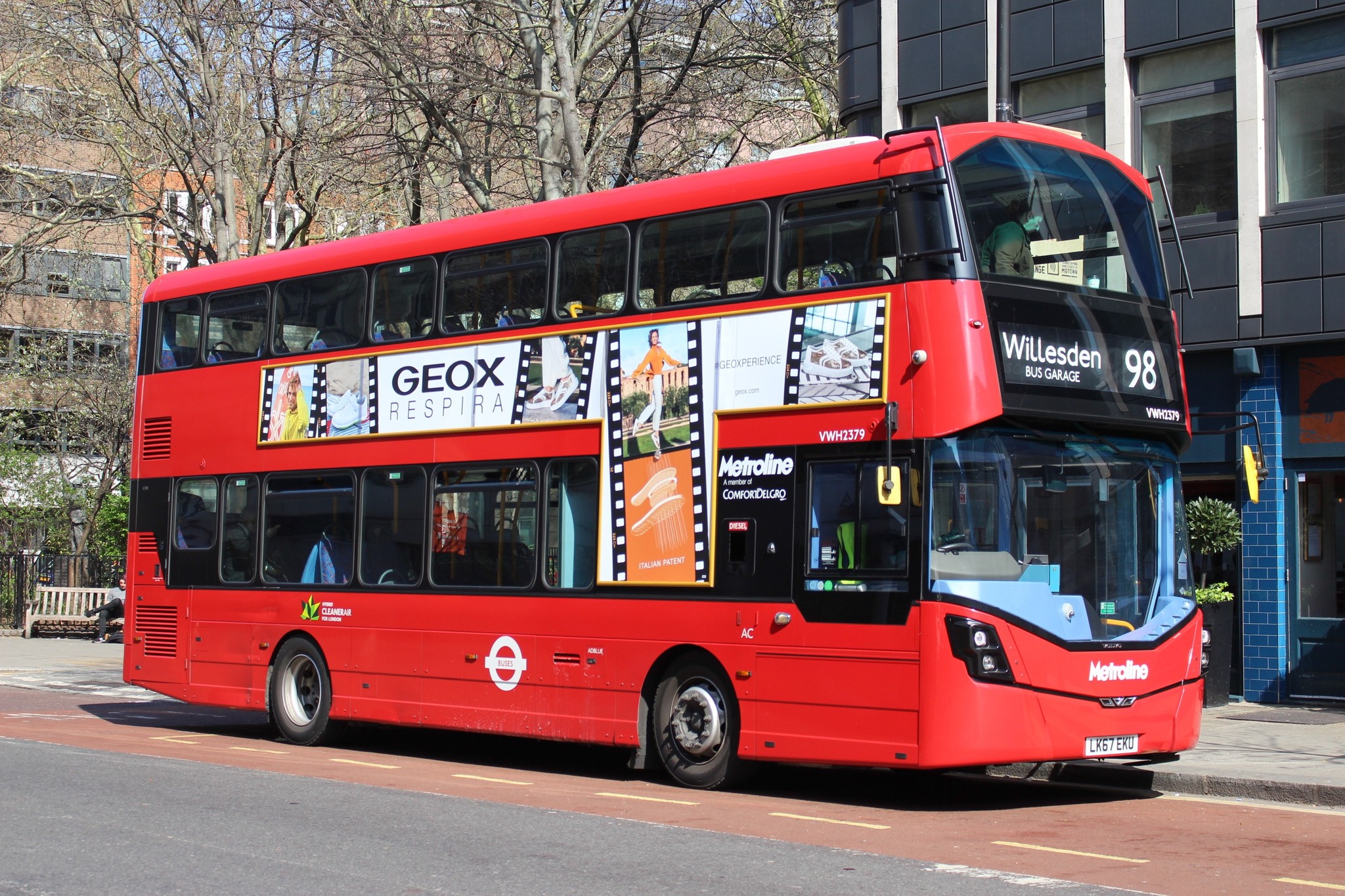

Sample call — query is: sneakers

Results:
[550,373,579,411]
[651,432,660,449]
[632,418,639,437]
[328,389,361,417]
[332,395,367,429]
[803,344,853,378]
[823,336,868,367]
[526,388,555,409]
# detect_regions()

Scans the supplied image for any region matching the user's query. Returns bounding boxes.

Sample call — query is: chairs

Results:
[320,523,407,585]
[454,515,533,587]
[159,303,534,368]
[818,259,895,288]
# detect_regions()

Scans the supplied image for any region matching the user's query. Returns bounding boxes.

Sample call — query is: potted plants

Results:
[1185,495,1244,708]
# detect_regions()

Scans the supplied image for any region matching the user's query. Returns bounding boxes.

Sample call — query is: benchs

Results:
[25,584,125,639]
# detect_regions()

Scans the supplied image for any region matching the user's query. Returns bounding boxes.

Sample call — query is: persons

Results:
[281,371,309,441]
[625,329,682,449]
[224,507,282,582]
[981,197,1045,279]
[84,575,126,643]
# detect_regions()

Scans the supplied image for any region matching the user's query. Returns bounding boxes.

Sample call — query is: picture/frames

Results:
[1304,522,1323,560]
[1304,479,1323,519]
